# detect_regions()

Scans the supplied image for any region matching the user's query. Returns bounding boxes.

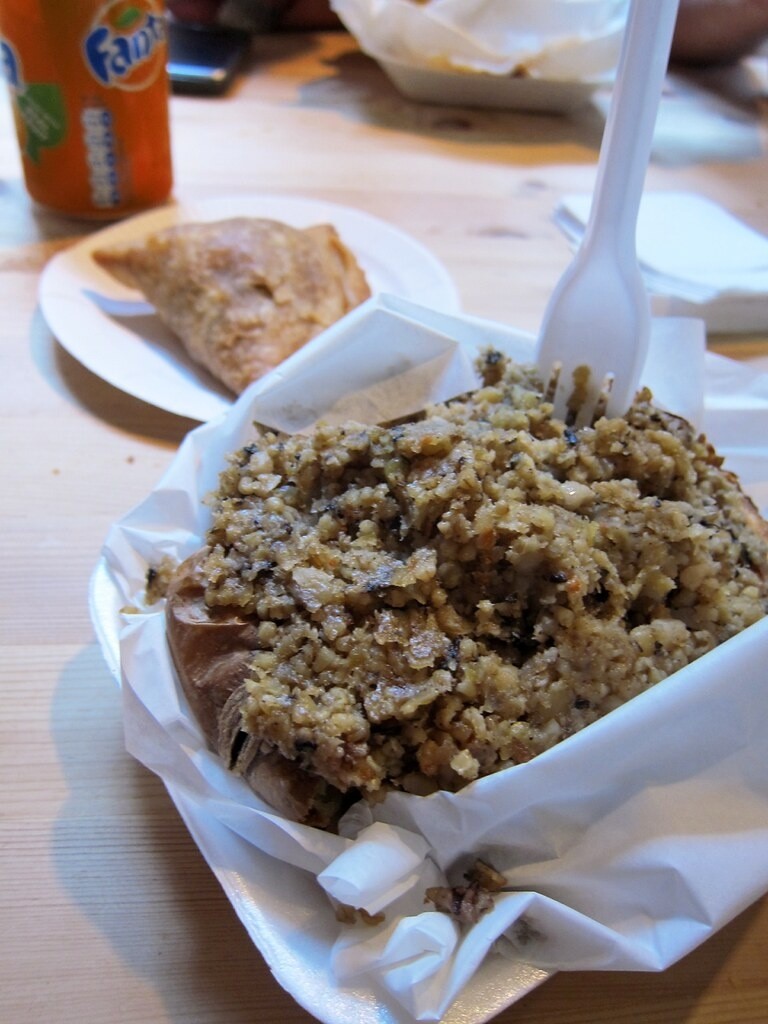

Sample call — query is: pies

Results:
[92,214,373,398]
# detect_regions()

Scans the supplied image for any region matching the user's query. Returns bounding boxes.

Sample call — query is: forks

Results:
[537,0,680,429]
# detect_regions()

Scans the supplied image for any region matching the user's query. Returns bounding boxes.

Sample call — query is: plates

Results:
[42,196,458,421]
[362,42,618,113]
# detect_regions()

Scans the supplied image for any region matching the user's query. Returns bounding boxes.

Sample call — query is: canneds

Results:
[0,0,174,220]
